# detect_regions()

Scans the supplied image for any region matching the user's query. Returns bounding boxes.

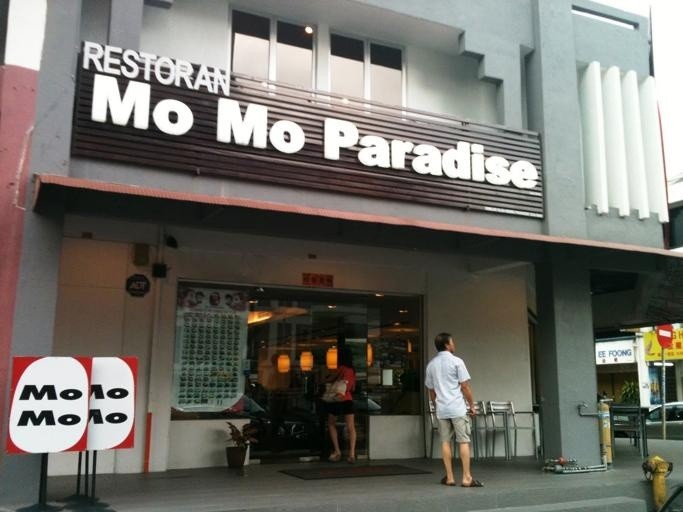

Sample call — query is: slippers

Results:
[461,477,483,487]
[441,475,455,486]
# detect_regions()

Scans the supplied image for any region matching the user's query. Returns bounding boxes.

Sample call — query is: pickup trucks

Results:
[597,394,649,438]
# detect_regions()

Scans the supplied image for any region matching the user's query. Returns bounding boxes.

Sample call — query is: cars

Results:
[639,402,683,440]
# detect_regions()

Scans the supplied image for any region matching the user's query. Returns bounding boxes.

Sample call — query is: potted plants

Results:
[222,421,259,469]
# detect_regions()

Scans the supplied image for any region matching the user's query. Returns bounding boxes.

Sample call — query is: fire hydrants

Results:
[643,455,672,512]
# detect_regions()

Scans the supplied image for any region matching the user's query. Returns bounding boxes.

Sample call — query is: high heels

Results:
[328,452,340,462]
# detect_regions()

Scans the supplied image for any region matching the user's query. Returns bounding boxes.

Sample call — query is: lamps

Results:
[277,343,374,374]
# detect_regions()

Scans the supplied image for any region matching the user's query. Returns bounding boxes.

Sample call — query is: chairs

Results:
[428,400,650,464]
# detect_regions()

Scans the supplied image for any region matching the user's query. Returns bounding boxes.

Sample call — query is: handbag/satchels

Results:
[321,366,349,402]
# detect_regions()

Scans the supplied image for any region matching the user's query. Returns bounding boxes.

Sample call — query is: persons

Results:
[424,333,477,487]
[318,347,359,465]
[243,398,270,449]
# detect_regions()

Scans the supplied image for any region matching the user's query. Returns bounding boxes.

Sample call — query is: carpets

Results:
[279,465,432,480]
